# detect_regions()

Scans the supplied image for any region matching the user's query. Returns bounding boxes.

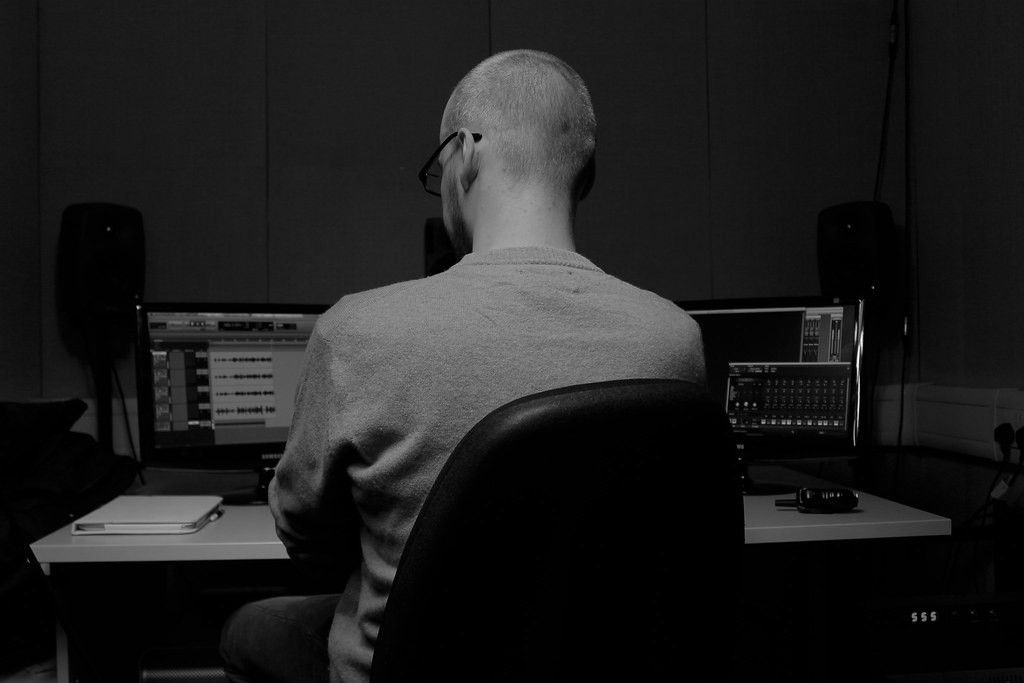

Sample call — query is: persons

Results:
[223,48,702,683]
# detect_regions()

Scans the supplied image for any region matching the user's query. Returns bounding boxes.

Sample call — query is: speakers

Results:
[819,201,908,353]
[55,202,146,363]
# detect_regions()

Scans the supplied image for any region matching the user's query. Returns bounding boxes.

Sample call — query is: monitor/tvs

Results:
[137,302,334,503]
[673,294,865,496]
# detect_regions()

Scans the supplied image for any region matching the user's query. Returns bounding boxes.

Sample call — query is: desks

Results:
[24,478,953,683]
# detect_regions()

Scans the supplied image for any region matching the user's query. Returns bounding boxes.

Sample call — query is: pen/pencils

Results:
[209,509,225,522]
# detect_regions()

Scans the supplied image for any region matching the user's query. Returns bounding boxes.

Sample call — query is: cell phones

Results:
[776,487,860,513]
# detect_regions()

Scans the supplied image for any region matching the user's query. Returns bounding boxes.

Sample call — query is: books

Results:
[72,495,223,535]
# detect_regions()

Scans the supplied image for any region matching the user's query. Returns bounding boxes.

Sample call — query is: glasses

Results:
[418,130,482,196]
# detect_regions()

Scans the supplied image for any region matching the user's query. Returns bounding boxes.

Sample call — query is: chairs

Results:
[367,379,747,683]
